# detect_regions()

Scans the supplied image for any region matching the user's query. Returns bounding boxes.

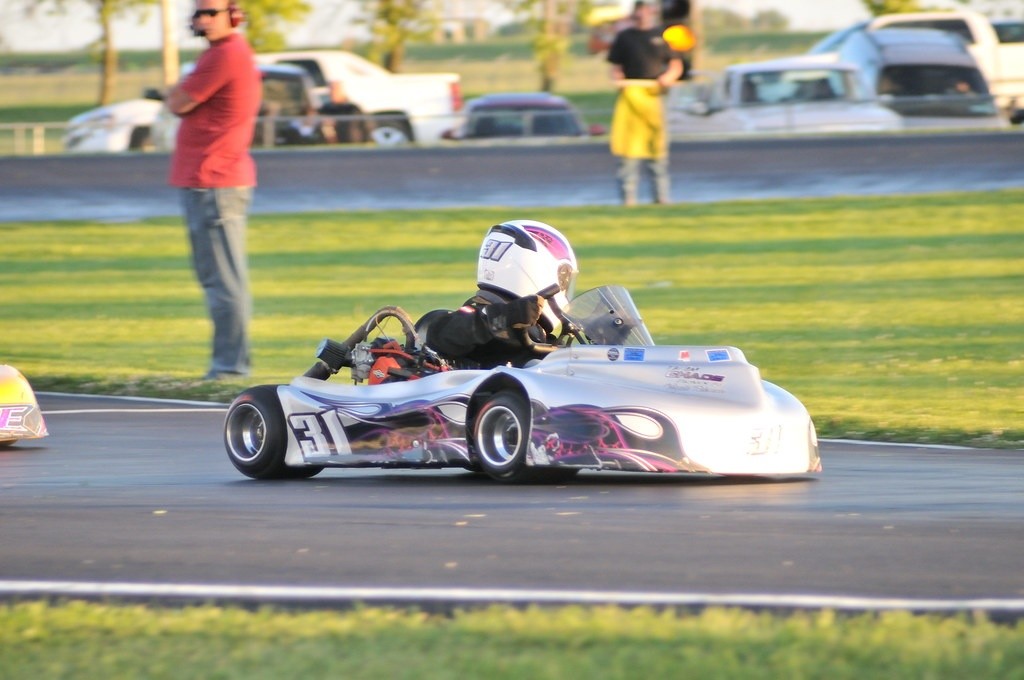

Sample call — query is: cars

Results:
[61,49,468,155]
[438,90,604,144]
[672,12,1023,134]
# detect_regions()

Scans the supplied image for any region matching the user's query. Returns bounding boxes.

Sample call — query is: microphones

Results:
[194,30,205,37]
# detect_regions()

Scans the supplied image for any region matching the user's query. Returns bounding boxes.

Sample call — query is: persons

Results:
[604,0,685,205]
[249,79,374,149]
[426,220,580,370]
[164,0,264,377]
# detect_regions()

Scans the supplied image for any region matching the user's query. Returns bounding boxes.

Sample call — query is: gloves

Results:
[484,295,545,338]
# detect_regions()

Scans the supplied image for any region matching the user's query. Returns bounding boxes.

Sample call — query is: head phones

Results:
[192,0,242,30]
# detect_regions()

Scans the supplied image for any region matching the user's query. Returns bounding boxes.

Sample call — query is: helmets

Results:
[477,217,575,336]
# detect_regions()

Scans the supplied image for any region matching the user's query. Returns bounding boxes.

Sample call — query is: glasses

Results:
[194,9,234,18]
